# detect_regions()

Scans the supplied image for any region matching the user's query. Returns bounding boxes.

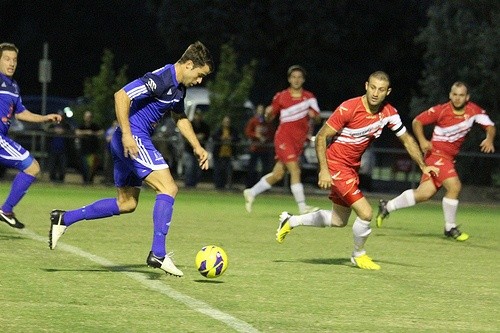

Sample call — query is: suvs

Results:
[304,109,374,191]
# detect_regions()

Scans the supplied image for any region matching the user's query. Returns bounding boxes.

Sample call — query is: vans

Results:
[166,87,256,178]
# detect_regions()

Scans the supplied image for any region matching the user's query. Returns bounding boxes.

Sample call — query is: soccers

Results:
[194,244,228,279]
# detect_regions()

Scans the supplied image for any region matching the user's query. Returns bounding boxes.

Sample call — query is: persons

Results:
[214,116,239,189]
[276,71,439,271]
[49,111,70,182]
[245,104,276,190]
[0,42,62,229]
[243,66,321,214]
[47,40,216,277]
[182,111,211,190]
[75,112,104,184]
[377,80,495,241]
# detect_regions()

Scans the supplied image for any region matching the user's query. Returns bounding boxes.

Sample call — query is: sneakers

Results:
[300,205,320,215]
[275,212,293,244]
[243,189,254,214]
[147,251,183,278]
[351,255,381,270]
[376,200,390,228]
[49,209,68,251]
[0,209,24,229]
[445,225,469,241]
